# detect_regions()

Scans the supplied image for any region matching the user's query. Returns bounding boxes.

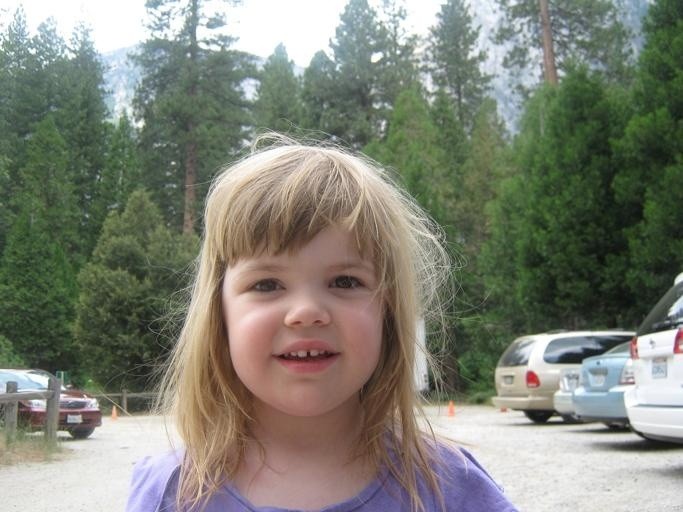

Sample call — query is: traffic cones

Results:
[110,405,117,423]
[448,400,455,415]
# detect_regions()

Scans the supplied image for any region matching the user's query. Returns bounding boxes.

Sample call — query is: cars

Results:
[1,367,102,440]
[492,272,683,446]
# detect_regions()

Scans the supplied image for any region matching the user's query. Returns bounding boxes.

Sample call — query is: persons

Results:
[120,131,521,512]
